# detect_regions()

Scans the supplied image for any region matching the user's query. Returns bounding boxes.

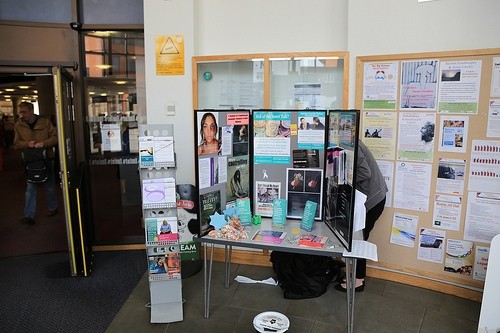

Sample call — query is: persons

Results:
[258,188,278,203]
[299,117,312,130]
[160,220,172,235]
[13,102,58,225]
[230,170,246,198]
[239,125,247,143]
[198,113,220,156]
[150,258,168,275]
[313,117,324,130]
[335,139,389,292]
[343,120,352,130]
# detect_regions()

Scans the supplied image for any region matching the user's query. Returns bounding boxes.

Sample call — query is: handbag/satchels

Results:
[25,164,48,184]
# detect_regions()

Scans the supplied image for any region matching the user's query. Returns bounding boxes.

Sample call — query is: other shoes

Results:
[334,274,366,292]
[47,207,59,217]
[20,216,35,225]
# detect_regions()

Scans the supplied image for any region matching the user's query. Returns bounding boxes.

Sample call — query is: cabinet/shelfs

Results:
[89,165,145,241]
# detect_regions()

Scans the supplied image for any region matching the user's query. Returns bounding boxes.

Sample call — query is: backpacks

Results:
[269,250,345,299]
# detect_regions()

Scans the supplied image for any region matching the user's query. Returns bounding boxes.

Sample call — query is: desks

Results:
[192,217,364,333]
